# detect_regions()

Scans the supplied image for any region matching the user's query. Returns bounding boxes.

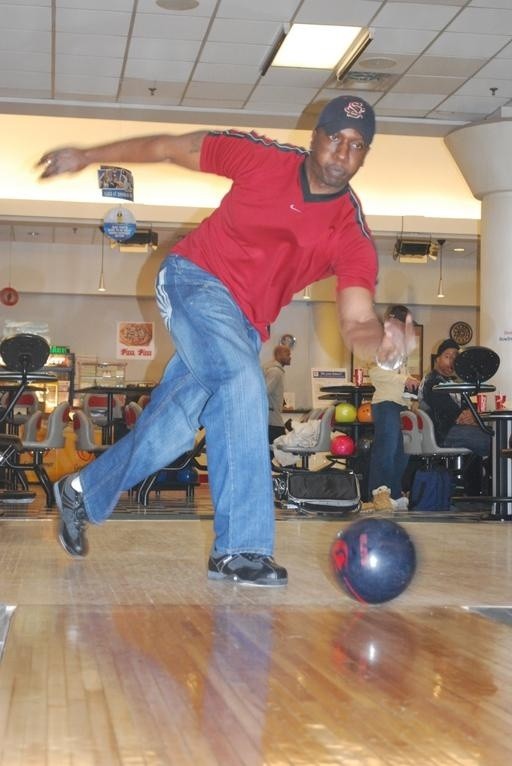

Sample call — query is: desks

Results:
[75,384,151,444]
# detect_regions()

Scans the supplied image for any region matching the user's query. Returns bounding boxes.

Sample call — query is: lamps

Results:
[97,228,107,292]
[394,238,437,264]
[435,240,446,297]
[118,229,158,253]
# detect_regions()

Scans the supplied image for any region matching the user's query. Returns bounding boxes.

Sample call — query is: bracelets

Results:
[374,354,404,372]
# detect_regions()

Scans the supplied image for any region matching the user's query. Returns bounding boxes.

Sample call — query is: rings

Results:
[401,351,406,355]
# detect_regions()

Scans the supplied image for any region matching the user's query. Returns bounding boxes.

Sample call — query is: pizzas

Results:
[120,324,152,346]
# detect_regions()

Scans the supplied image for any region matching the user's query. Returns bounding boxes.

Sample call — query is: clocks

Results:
[450,321,472,346]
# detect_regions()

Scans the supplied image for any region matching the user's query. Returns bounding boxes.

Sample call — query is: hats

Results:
[436,338,461,356]
[317,94,375,144]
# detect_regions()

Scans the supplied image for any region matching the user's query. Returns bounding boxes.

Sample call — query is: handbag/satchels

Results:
[408,462,452,512]
[283,471,362,517]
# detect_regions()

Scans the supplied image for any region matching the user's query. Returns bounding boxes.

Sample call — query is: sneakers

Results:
[360,493,394,513]
[207,551,289,588]
[53,471,89,557]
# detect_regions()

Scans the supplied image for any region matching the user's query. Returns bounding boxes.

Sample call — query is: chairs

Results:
[0,402,336,509]
[399,408,474,512]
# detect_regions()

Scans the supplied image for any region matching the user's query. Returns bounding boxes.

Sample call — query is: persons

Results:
[33,97,420,589]
[417,339,492,505]
[262,345,292,471]
[369,306,420,510]
